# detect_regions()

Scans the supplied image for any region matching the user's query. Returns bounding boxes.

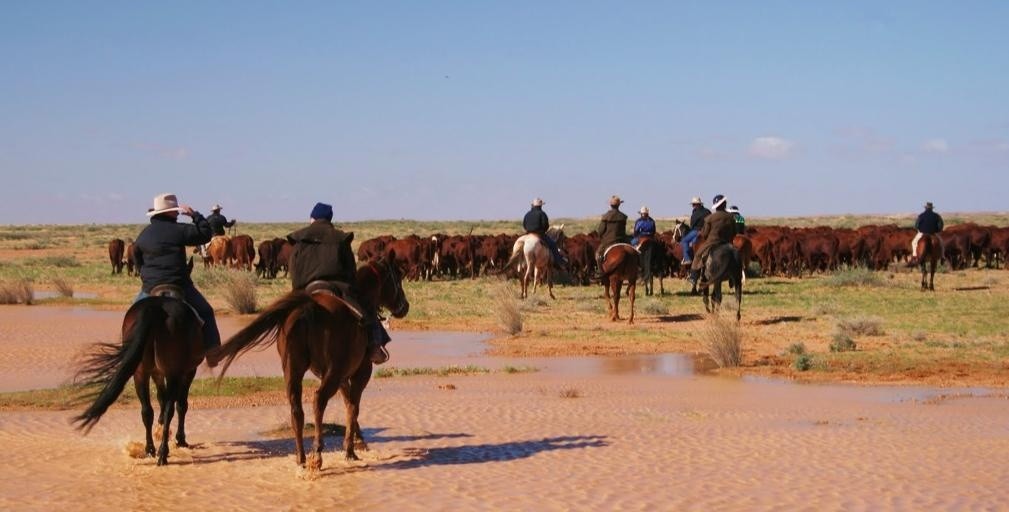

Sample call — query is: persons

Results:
[130,193,229,367]
[594,196,628,279]
[523,199,568,265]
[201,204,236,259]
[631,207,656,244]
[287,202,388,364]
[911,201,946,266]
[679,195,744,284]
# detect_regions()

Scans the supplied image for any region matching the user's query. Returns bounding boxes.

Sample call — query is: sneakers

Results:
[207,354,222,367]
[368,352,384,362]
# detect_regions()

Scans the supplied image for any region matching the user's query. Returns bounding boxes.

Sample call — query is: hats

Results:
[689,197,703,204]
[146,195,188,216]
[638,207,649,214]
[924,201,936,207]
[609,195,623,206]
[530,198,544,207]
[311,202,332,221]
[210,204,223,211]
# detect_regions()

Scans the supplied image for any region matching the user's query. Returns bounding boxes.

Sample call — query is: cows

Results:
[107,235,144,277]
[232,231,296,280]
[358,221,1008,286]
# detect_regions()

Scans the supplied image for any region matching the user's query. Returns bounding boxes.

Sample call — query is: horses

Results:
[885,229,941,291]
[69,255,206,467]
[209,246,410,472]
[490,223,569,302]
[193,233,230,279]
[592,233,671,325]
[671,220,743,321]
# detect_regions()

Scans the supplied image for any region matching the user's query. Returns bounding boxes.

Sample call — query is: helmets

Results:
[713,195,726,209]
